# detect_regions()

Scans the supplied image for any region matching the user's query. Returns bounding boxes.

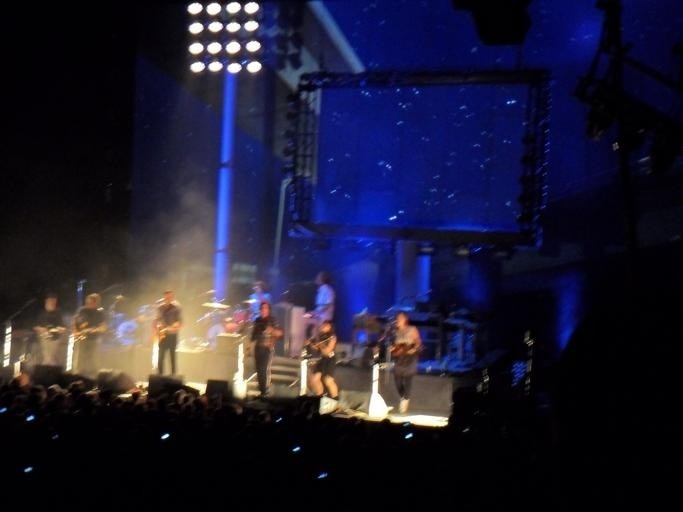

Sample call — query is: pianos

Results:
[284,306,318,358]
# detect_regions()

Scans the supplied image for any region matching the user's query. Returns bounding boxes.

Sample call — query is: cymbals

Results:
[201,303,232,308]
[240,300,260,304]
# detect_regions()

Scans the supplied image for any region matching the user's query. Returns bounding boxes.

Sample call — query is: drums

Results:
[208,321,225,348]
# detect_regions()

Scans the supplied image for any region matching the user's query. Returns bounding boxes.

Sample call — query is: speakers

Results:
[33,364,60,386]
[206,380,233,396]
[268,394,341,419]
[98,369,137,392]
[148,376,198,399]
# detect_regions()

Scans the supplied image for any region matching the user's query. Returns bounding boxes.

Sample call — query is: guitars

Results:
[156,321,181,342]
[75,322,106,344]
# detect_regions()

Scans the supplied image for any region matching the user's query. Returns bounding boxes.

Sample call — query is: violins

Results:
[319,331,333,343]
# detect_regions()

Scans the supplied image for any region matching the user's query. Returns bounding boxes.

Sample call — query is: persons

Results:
[2,372,484,512]
[33,289,183,376]
[209,271,421,413]
